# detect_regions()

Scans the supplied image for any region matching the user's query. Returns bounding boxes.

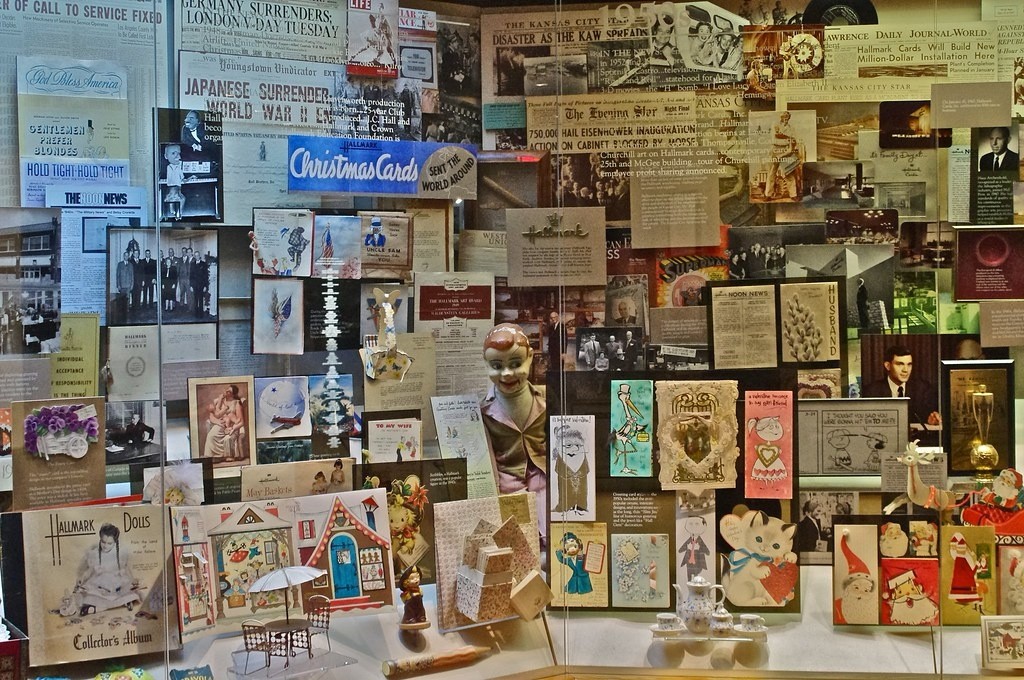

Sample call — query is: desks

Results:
[264,618,311,665]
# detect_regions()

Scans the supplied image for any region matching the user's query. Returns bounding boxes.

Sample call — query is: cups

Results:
[740,614,765,631]
[656,613,681,629]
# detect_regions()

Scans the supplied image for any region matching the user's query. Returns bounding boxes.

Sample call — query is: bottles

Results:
[710,602,733,637]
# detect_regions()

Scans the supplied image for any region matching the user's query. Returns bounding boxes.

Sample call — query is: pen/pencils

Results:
[380,642,496,677]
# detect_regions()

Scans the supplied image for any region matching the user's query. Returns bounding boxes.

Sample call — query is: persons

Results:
[2,307,44,326]
[649,14,802,201]
[399,564,426,625]
[861,346,942,427]
[116,246,208,319]
[479,321,546,571]
[728,244,786,279]
[123,413,155,444]
[979,128,1019,172]
[342,4,527,146]
[548,153,638,370]
[309,458,348,496]
[78,523,142,617]
[856,277,872,329]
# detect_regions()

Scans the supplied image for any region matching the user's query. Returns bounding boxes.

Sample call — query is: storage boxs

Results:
[510,569,554,622]
[453,574,514,623]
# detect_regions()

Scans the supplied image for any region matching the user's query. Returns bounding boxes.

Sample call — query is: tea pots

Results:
[672,574,725,633]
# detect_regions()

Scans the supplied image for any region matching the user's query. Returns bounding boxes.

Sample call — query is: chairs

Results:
[240,619,290,679]
[289,595,332,657]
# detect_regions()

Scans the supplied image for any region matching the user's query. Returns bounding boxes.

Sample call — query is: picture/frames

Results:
[186,374,257,478]
[103,226,220,363]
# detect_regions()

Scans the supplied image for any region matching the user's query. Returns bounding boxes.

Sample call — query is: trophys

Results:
[969,384,998,482]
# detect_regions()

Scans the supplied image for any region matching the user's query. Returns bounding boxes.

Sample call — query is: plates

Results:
[734,624,768,633]
[650,624,686,634]
